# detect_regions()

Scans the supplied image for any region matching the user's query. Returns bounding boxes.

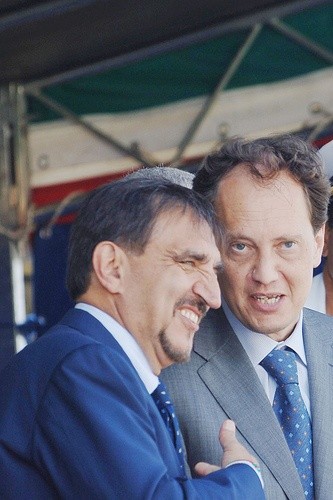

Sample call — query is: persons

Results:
[159,133,333,500]
[0,177,265,500]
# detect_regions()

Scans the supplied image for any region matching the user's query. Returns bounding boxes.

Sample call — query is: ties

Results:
[259,347,315,500]
[149,380,188,480]
